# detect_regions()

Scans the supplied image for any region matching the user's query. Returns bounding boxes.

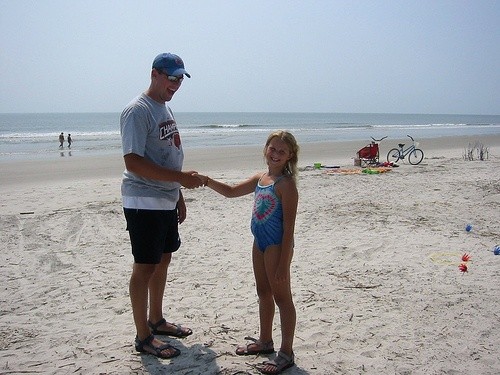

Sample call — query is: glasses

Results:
[159,71,184,82]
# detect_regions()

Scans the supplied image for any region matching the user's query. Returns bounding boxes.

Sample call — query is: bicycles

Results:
[387,135,424,165]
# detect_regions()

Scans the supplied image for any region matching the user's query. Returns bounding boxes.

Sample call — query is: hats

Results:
[152,52,191,78]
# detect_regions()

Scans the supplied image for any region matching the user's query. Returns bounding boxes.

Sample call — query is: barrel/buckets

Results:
[314,163,321,169]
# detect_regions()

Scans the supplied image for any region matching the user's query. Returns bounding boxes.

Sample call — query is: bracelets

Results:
[204,176,209,187]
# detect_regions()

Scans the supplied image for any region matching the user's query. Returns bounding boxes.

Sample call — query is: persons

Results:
[120,52,203,359]
[59,132,64,147]
[191,130,299,375]
[67,134,72,147]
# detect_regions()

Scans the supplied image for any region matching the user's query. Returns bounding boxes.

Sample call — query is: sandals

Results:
[147,319,192,337]
[261,351,294,375]
[236,340,274,355]
[135,334,180,358]
